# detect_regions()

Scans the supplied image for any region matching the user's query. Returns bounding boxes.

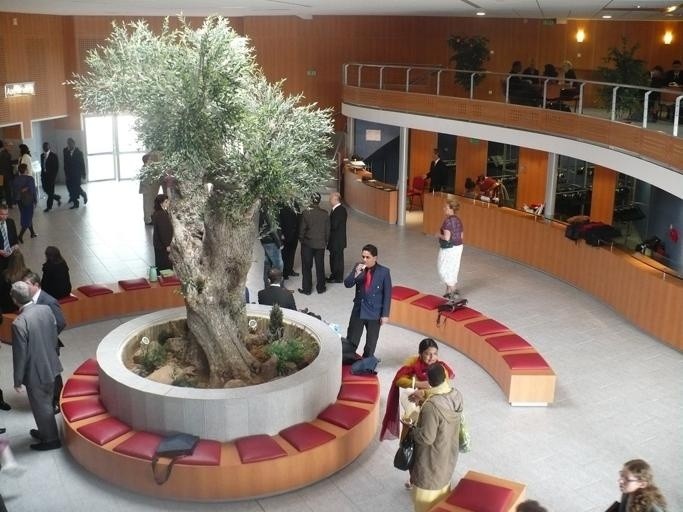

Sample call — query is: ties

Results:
[365,270,372,290]
[1,224,11,250]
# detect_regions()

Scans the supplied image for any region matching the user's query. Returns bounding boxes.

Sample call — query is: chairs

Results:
[498,75,581,114]
[657,87,682,121]
[407,177,426,211]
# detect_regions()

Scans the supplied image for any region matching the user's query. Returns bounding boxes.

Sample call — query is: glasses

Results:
[619,471,639,483]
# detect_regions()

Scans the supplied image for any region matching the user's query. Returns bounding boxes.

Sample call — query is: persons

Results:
[503,58,683,122]
[342,243,393,358]
[461,175,510,210]
[422,147,450,192]
[614,457,668,512]
[0,137,88,452]
[434,193,465,299]
[390,335,452,493]
[515,498,550,512]
[395,361,464,512]
[150,190,349,312]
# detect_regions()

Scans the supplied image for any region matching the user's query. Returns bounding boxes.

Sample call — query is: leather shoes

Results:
[56,195,61,207]
[284,270,343,295]
[69,191,87,209]
[53,402,60,413]
[0,402,11,410]
[29,429,46,441]
[0,427,6,434]
[43,206,52,211]
[18,236,24,243]
[30,233,38,238]
[31,440,61,450]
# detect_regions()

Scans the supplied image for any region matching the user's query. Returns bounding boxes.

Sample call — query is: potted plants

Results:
[598,35,653,125]
[56,11,343,444]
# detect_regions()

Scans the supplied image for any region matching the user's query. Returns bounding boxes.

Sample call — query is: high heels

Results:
[444,293,452,299]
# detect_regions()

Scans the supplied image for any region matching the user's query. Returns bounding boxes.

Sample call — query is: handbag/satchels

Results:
[393,425,416,470]
[439,239,453,248]
[21,188,34,209]
[459,426,471,453]
[156,434,198,456]
[438,298,467,311]
[351,356,381,376]
